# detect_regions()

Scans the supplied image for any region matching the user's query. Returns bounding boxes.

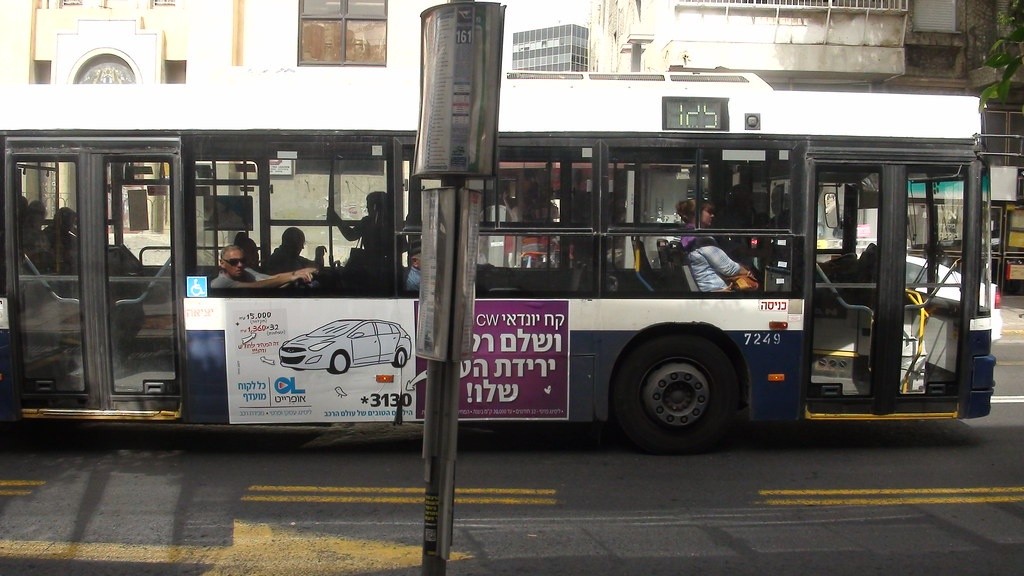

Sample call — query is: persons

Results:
[21,197,78,274]
[720,186,773,266]
[406,247,421,291]
[328,192,393,267]
[264,227,326,274]
[211,245,319,288]
[689,234,758,293]
[238,238,258,265]
[569,191,591,264]
[676,199,714,246]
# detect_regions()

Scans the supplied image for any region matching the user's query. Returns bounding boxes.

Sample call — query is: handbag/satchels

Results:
[343,248,367,278]
[736,275,758,289]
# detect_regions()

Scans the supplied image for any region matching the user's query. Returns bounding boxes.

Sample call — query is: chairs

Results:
[657,239,698,292]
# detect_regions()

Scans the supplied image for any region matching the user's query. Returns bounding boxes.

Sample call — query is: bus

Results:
[0,72,1024,455]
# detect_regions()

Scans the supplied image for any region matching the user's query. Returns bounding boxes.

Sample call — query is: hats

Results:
[521,244,547,257]
[410,240,421,257]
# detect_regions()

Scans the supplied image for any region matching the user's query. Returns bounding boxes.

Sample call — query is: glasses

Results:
[224,259,246,265]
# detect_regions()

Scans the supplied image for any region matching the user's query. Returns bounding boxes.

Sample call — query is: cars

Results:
[905,257,1003,342]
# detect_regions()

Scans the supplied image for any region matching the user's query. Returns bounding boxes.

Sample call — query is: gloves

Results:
[746,270,759,282]
